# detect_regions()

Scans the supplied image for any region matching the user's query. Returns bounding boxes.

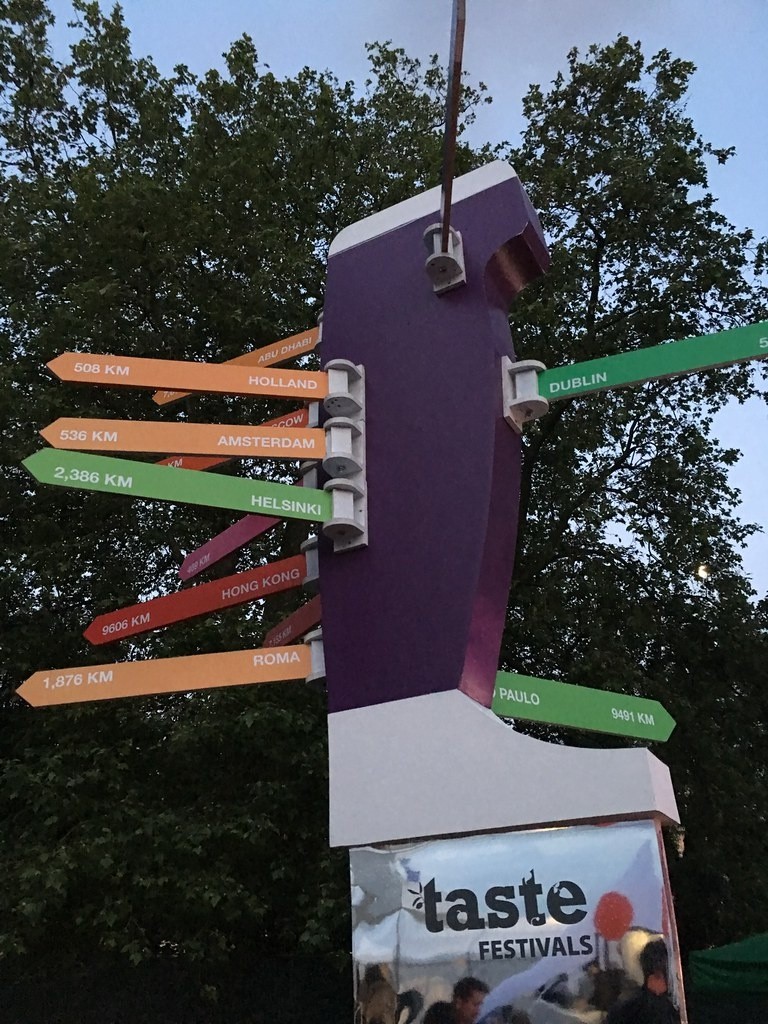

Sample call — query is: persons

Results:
[353,937,686,1024]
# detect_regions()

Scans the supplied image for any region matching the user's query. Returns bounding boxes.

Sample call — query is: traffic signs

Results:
[39,417,329,461]
[491,669,677,743]
[46,352,329,400]
[20,448,334,526]
[153,326,319,406]
[153,401,321,471]
[83,548,319,644]
[263,594,322,647]
[16,640,326,707]
[516,321,768,400]
[422,0,468,299]
[178,466,318,583]
